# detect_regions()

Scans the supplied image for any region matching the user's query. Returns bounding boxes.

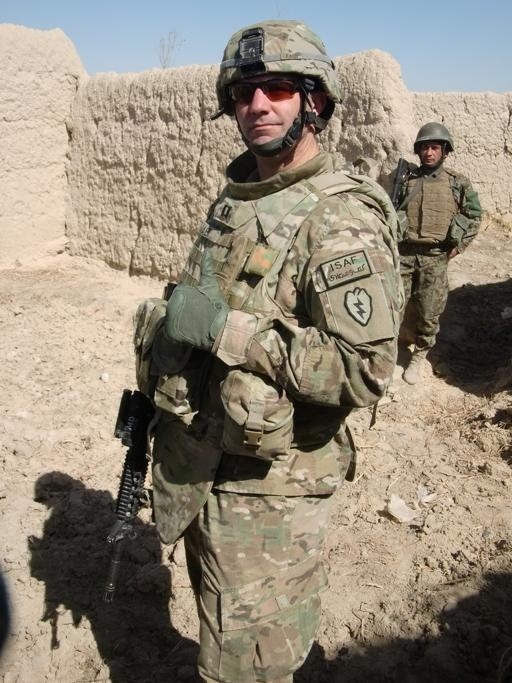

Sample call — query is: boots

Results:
[403,344,431,384]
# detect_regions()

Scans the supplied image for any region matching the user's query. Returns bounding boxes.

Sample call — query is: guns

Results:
[391,158,409,209]
[102,281,176,604]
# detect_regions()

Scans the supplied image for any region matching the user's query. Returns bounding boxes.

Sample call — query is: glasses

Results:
[224,77,318,103]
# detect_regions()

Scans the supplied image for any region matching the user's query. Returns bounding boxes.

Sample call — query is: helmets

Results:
[216,22,340,133]
[414,123,455,155]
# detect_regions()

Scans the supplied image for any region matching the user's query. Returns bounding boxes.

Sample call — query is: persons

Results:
[392,121,482,385]
[127,16,405,683]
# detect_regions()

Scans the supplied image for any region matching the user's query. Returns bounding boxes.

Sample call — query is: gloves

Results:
[162,255,229,351]
